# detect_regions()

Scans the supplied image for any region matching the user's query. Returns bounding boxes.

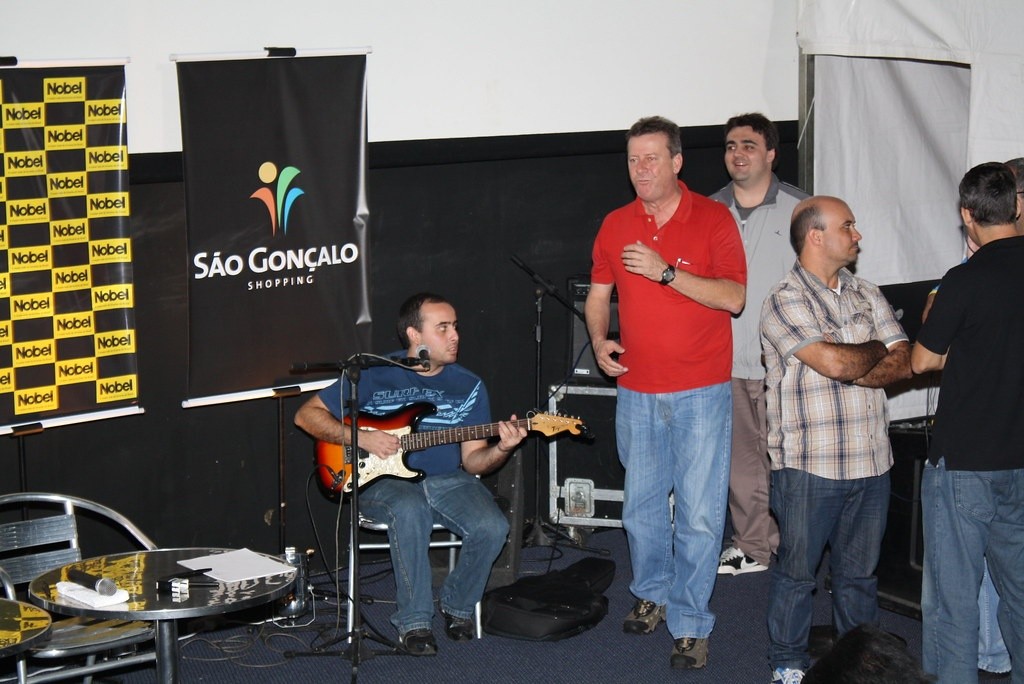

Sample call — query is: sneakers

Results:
[669,637,709,670]
[717,546,769,576]
[398,627,438,657]
[771,666,804,684]
[439,606,476,642]
[623,598,666,635]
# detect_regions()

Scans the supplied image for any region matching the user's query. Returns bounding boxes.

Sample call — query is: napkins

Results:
[56,581,130,608]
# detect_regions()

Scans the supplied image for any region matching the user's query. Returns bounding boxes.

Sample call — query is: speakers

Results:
[566,274,621,386]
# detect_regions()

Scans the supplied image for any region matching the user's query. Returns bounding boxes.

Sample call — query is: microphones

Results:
[67,570,117,596]
[416,344,431,371]
[607,331,620,340]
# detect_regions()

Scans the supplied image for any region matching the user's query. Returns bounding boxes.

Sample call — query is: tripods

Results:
[510,255,586,547]
[283,357,422,684]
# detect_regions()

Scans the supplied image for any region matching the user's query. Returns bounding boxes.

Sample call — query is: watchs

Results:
[660,265,676,286]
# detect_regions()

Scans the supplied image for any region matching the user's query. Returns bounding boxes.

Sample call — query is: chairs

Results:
[346,508,486,643]
[0,493,181,684]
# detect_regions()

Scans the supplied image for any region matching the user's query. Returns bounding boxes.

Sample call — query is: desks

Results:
[28,548,298,684]
[0,597,50,657]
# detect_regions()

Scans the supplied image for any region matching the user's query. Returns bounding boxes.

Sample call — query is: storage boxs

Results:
[549,385,679,531]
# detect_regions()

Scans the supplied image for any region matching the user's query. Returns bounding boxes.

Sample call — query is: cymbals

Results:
[0,595,54,658]
[26,545,300,621]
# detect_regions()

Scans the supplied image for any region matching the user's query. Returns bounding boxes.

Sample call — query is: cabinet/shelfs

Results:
[869,424,932,622]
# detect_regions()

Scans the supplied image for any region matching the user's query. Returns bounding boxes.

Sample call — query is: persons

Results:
[800,622,939,684]
[911,157,1024,683]
[704,112,814,579]
[760,196,913,684]
[294,291,528,656]
[584,114,747,670]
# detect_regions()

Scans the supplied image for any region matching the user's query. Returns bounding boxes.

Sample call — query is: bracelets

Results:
[497,443,513,453]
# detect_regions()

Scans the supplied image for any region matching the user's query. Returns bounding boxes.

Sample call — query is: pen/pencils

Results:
[188,581,219,587]
[172,566,212,577]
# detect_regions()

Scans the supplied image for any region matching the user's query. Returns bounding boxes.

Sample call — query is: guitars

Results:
[312,399,598,500]
